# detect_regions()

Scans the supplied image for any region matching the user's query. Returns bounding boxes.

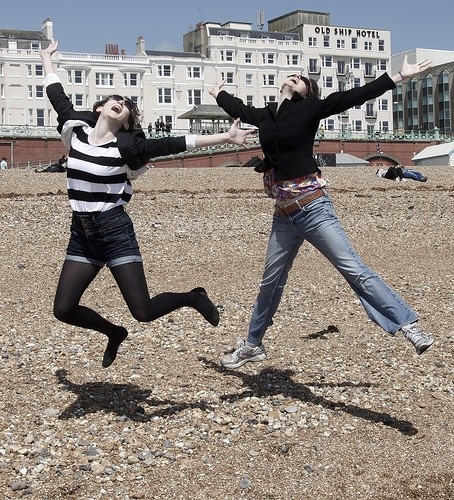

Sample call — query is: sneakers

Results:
[402,323,435,356]
[220,338,267,368]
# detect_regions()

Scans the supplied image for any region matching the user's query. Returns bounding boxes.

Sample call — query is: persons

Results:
[207,54,435,368]
[148,119,172,138]
[319,124,324,137]
[35,154,67,173]
[376,164,428,182]
[39,37,258,368]
[0,157,8,170]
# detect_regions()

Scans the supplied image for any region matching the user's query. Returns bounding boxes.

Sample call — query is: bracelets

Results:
[398,70,405,81]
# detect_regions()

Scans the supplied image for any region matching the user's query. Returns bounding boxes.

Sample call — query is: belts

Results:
[276,188,325,217]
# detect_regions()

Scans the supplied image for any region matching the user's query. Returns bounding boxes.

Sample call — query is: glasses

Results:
[113,94,132,111]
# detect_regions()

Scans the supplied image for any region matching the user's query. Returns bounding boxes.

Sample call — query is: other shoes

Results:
[102,326,128,368]
[189,287,219,327]
